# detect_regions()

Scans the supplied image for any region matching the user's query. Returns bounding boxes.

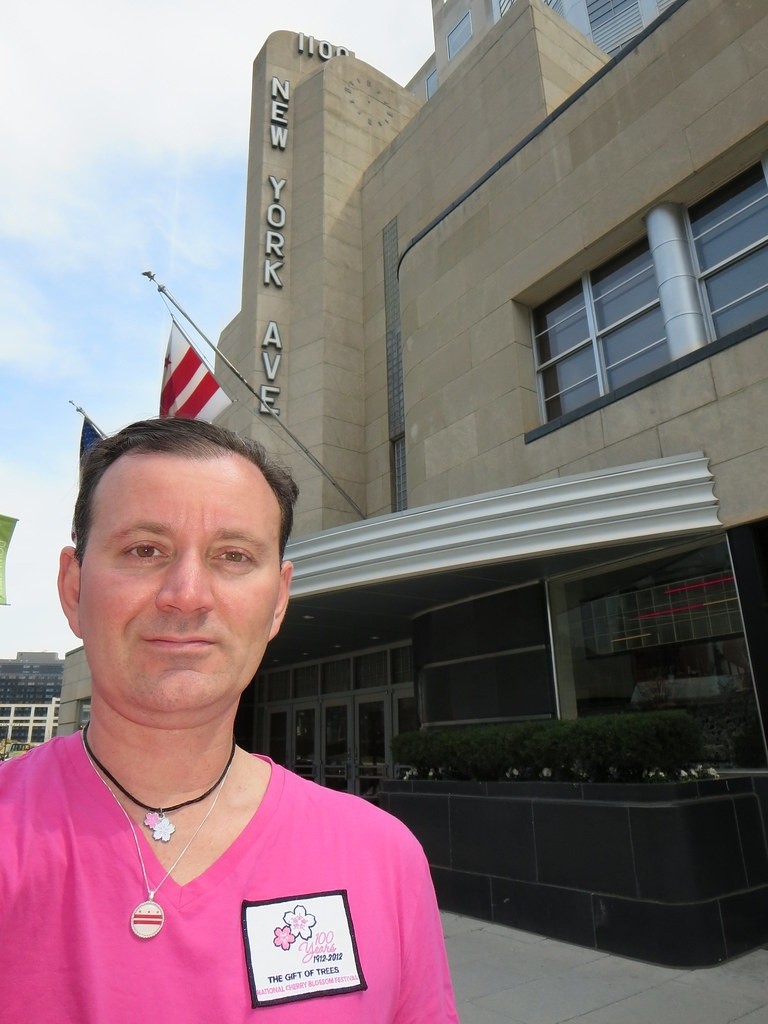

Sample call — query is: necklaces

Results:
[78,721,236,938]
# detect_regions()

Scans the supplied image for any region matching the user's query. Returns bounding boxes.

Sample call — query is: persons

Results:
[2,417,460,1024]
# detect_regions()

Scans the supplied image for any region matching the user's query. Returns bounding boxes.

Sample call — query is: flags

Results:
[159,321,232,424]
[80,420,103,461]
